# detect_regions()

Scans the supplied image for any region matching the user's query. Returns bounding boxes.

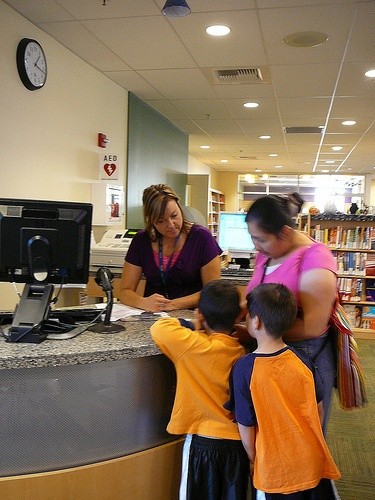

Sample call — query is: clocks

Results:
[16,38,47,91]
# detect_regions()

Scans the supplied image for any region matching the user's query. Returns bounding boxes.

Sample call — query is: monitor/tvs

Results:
[216,211,259,268]
[0,197,92,343]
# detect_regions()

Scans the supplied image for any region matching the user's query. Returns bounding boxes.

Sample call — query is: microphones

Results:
[96,268,114,304]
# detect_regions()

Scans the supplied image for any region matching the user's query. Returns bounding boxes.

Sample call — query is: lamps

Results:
[161,0,191,18]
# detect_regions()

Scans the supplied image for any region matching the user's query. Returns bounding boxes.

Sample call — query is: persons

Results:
[119,184,222,315]
[232,282,341,500]
[150,280,250,500]
[244,192,339,446]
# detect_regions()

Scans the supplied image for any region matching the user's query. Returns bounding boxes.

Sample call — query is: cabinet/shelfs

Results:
[187,173,225,240]
[299,214,375,340]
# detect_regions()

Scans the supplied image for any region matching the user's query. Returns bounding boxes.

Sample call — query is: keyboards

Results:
[0,308,106,324]
[219,268,254,278]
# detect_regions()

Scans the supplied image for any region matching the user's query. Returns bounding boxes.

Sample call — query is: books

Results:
[207,191,225,239]
[300,223,375,304]
